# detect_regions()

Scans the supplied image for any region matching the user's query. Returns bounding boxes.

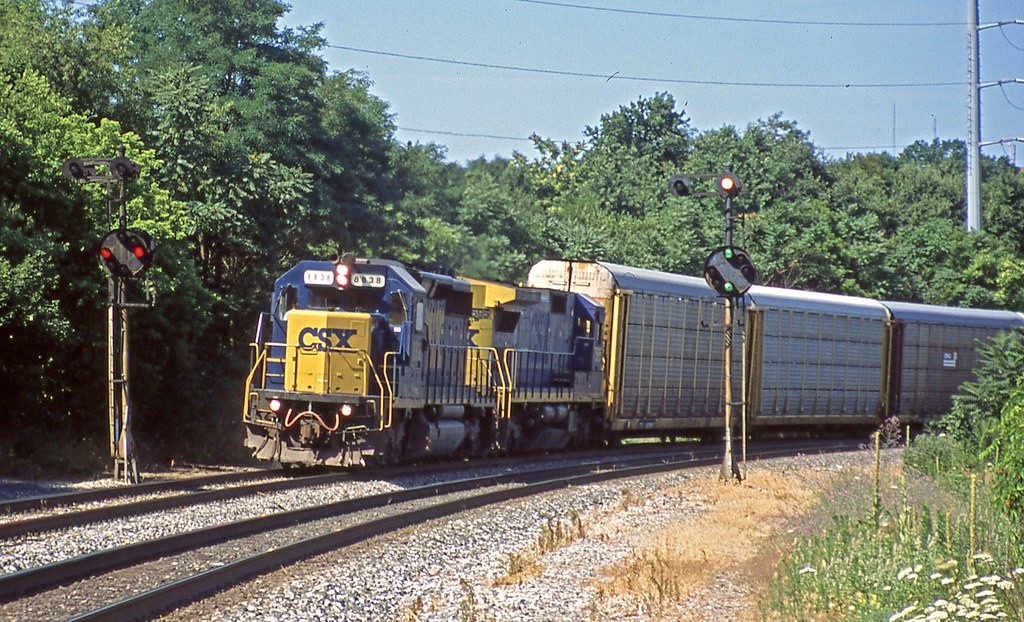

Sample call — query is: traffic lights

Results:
[63,156,143,181]
[668,174,741,197]
[99,228,151,276]
[704,244,756,296]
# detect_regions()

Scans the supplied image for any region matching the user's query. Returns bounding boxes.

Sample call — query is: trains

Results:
[243,250,1024,474]
